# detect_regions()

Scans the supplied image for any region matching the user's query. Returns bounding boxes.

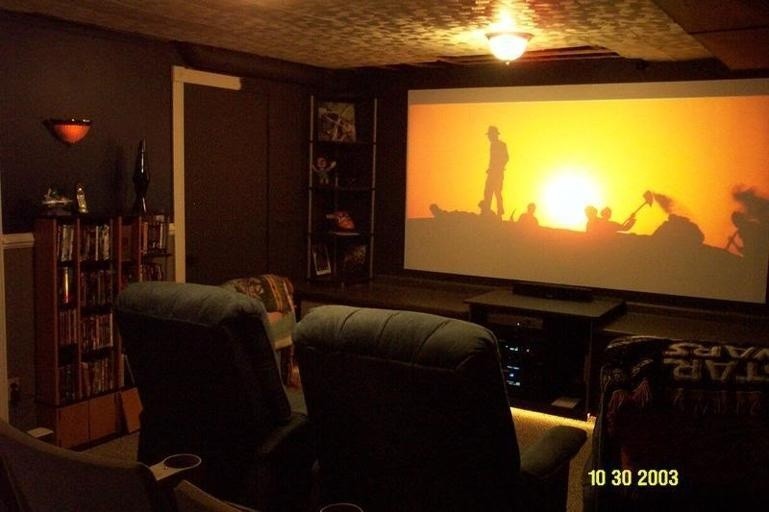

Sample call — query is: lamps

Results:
[484,32,534,66]
[48,117,92,146]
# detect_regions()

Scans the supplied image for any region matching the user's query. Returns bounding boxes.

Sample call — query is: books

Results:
[57,215,169,403]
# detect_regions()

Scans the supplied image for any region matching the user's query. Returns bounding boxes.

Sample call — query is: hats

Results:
[484,125,501,136]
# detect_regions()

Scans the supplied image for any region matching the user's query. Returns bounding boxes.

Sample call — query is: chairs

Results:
[112,280,319,512]
[290,304,587,512]
[581,336,768,512]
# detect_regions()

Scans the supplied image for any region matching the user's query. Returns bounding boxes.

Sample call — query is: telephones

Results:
[72,182,90,215]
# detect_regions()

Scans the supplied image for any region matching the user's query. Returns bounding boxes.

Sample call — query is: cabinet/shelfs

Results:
[463,289,626,415]
[307,95,378,298]
[32,210,171,449]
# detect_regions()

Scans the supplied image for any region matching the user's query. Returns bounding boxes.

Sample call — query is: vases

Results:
[131,136,150,217]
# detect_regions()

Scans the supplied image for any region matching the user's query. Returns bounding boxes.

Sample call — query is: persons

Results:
[311,156,337,184]
[584,206,621,232]
[480,124,510,222]
[518,203,539,226]
[478,200,496,222]
[599,208,637,234]
[429,203,476,218]
[728,210,768,260]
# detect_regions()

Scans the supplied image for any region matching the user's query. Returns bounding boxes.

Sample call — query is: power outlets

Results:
[7,377,20,401]
